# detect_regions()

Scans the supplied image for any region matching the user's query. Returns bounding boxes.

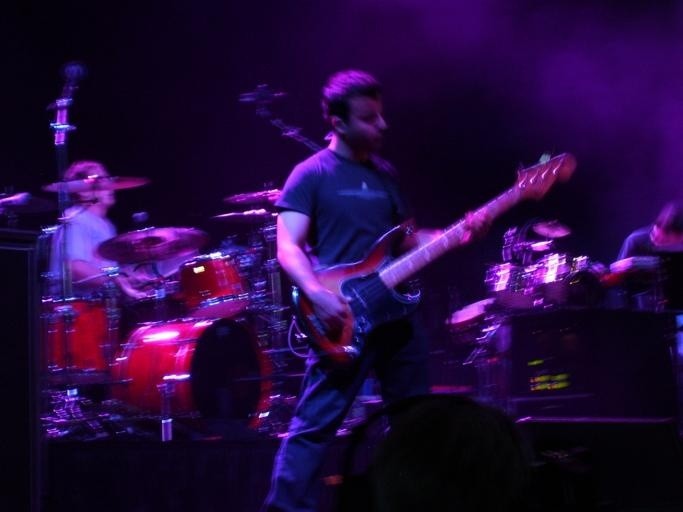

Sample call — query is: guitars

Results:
[292,149,579,368]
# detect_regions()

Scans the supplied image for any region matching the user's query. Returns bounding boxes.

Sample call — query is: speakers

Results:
[506,414,682,507]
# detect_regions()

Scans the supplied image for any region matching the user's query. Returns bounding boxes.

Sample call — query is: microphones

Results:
[52,63,82,147]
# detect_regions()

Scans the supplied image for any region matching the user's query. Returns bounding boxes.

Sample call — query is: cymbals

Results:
[0,193,53,211]
[47,177,144,193]
[226,188,283,204]
[96,228,207,266]
[218,208,278,221]
[534,222,572,240]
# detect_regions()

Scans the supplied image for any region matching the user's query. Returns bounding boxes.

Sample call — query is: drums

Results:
[171,251,249,319]
[444,298,501,347]
[254,261,289,312]
[109,317,271,446]
[531,254,574,305]
[38,295,120,390]
[488,260,535,317]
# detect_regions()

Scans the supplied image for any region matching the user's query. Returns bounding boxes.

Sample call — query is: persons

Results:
[254,66,496,510]
[47,159,187,341]
[613,197,682,269]
